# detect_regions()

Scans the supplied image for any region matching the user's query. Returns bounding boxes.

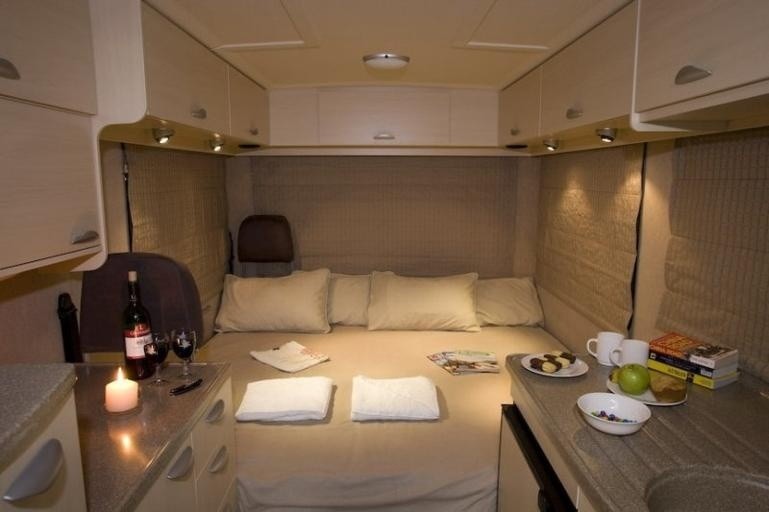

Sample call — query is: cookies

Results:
[533,349,576,375]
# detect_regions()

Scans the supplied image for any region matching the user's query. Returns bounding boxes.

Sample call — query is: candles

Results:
[104,367,139,412]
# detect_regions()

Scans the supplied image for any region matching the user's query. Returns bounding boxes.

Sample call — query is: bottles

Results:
[120,270,157,381]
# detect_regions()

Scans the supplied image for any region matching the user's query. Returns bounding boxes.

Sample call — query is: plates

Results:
[604,375,689,408]
[520,355,590,381]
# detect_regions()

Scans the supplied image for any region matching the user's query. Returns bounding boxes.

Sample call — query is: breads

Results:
[650,376,687,404]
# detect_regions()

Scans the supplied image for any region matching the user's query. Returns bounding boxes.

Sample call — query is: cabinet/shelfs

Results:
[0,360,87,512]
[138,3,268,146]
[266,86,501,155]
[74,366,237,512]
[2,2,104,282]
[505,3,769,159]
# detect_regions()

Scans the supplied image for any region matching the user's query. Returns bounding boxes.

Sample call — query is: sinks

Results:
[644,463,769,512]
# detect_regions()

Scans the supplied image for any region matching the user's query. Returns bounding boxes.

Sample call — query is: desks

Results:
[496,351,769,512]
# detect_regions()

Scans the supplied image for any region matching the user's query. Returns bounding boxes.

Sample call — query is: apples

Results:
[617,363,648,395]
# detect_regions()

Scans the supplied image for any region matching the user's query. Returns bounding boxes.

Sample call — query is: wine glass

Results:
[142,327,175,392]
[172,328,205,383]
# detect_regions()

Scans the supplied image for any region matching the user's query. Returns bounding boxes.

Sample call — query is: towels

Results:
[235,376,332,421]
[351,376,440,422]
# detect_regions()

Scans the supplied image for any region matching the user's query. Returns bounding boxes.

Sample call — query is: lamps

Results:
[208,139,224,153]
[152,127,175,146]
[543,140,559,152]
[596,128,617,143]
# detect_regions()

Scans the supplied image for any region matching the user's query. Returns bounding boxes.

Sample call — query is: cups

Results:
[609,336,650,372]
[587,327,623,367]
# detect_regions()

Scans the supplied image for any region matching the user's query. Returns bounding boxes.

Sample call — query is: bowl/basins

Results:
[573,390,648,435]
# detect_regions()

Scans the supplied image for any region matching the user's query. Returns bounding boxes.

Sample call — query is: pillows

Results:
[367,270,481,332]
[214,270,331,333]
[475,277,544,327]
[327,272,370,326]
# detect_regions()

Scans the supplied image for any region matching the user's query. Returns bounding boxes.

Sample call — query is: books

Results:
[426,347,503,375]
[647,331,740,390]
[250,340,329,374]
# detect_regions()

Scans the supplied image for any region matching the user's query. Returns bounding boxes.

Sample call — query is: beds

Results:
[187,325,573,512]
